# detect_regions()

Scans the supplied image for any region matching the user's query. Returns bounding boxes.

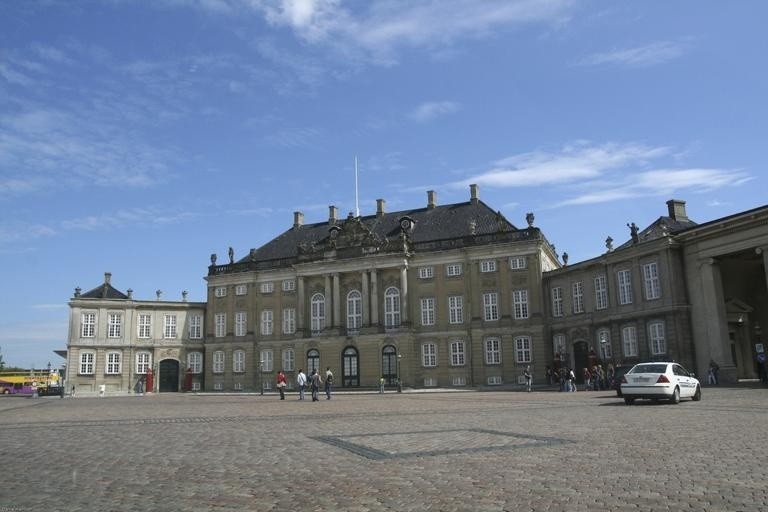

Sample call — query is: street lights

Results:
[259,359,264,395]
[46,361,51,397]
[396,352,402,392]
[600,336,607,391]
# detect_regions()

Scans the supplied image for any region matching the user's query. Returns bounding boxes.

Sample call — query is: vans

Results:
[613,361,639,397]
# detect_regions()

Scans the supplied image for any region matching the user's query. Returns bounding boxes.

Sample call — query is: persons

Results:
[397,377,402,392]
[296,369,306,400]
[707,359,719,385]
[757,352,768,385]
[325,366,333,399]
[546,362,577,392]
[99,383,105,397]
[524,365,532,392]
[71,384,76,397]
[583,362,615,391]
[277,371,286,400]
[380,377,385,394]
[309,369,323,402]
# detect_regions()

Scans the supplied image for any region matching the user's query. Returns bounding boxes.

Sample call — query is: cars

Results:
[619,361,701,404]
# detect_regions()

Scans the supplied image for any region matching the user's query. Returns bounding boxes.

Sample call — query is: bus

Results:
[0,372,61,395]
